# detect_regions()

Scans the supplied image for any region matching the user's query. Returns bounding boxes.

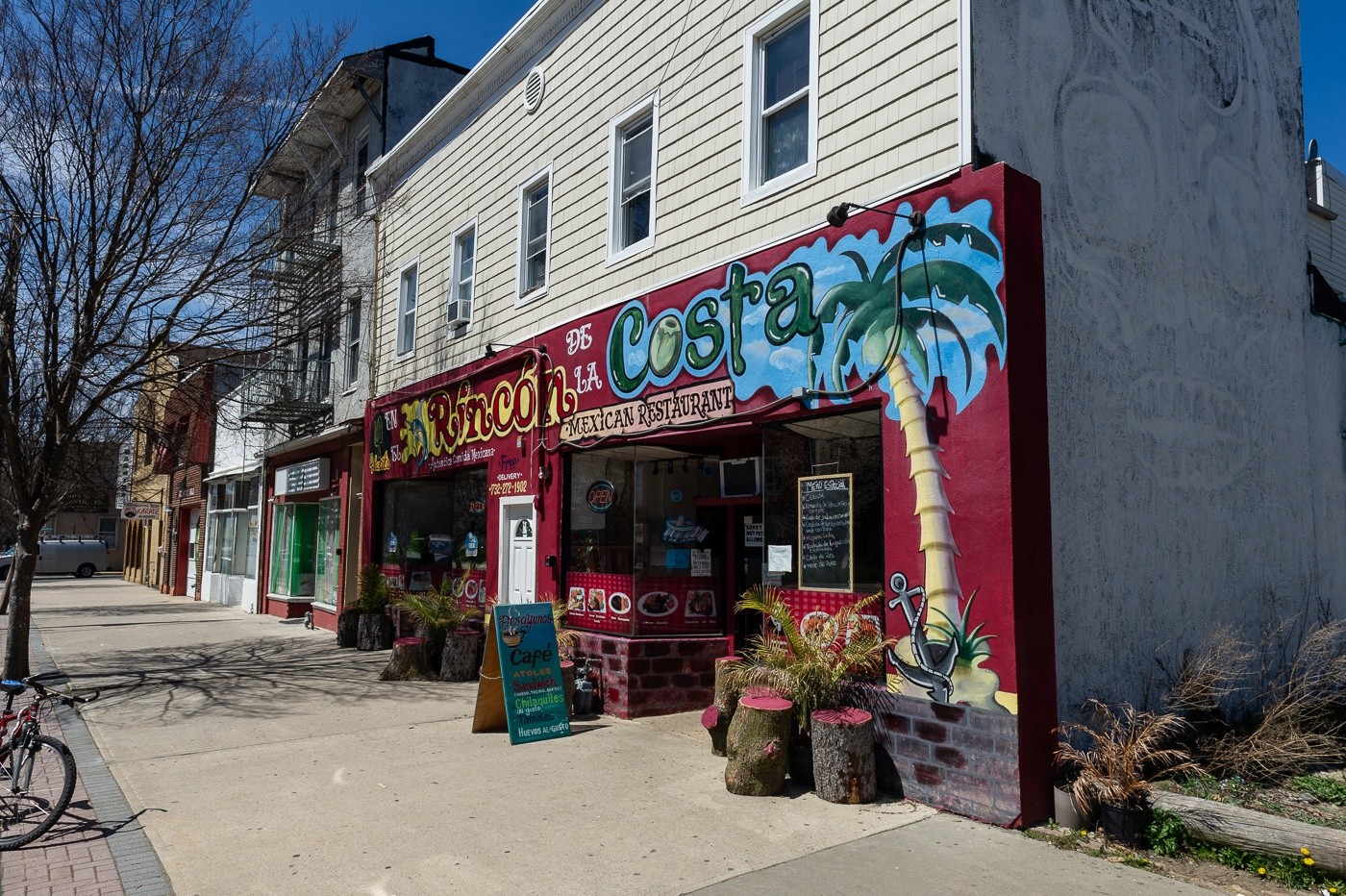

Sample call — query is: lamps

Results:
[485,342,546,360]
[825,436,860,460]
[651,457,705,476]
[826,202,925,229]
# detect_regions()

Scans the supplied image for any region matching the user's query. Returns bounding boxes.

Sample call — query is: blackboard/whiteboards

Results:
[795,472,856,595]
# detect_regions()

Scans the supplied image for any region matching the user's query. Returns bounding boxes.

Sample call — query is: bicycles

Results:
[1,669,103,854]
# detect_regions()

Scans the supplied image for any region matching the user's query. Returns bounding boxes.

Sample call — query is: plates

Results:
[452,578,463,598]
[567,586,585,612]
[478,580,486,603]
[845,614,881,649]
[609,593,631,614]
[684,590,717,617]
[588,588,606,613]
[800,612,839,647]
[638,591,678,616]
[464,579,478,599]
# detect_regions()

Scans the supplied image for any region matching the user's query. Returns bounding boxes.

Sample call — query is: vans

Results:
[0,533,111,581]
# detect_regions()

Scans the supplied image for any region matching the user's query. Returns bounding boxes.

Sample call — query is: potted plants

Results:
[714,582,900,786]
